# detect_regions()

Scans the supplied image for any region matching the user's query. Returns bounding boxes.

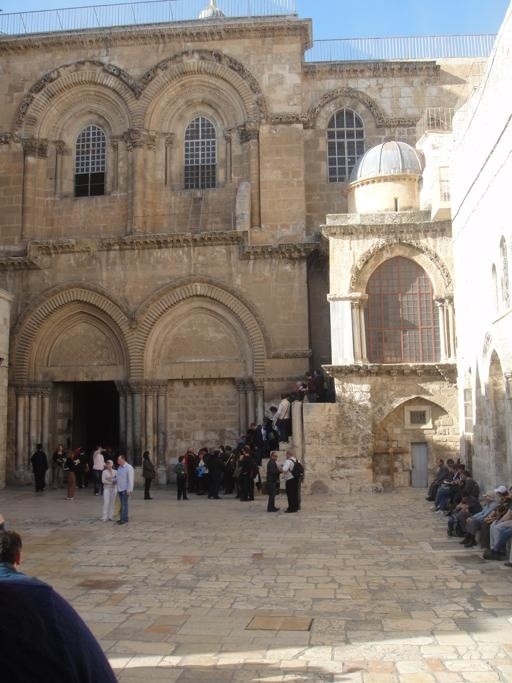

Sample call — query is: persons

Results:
[53,444,115,500]
[116,454,134,525]
[143,452,156,500]
[31,444,47,493]
[0,530,119,683]
[174,369,333,513]
[425,458,512,567]
[101,459,117,522]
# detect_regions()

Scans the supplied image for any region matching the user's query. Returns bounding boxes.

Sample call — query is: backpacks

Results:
[288,458,304,479]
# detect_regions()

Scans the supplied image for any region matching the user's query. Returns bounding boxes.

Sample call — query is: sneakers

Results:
[460,535,509,561]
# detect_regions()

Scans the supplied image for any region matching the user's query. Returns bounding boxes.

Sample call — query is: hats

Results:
[482,491,495,501]
[495,485,508,494]
